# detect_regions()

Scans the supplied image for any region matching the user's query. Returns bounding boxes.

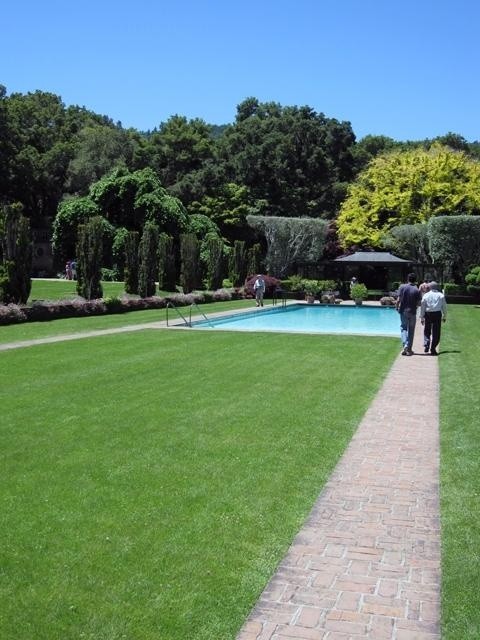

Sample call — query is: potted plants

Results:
[303,281,317,304]
[350,283,369,305]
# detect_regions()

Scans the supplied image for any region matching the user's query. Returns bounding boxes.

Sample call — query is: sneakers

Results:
[402,346,439,355]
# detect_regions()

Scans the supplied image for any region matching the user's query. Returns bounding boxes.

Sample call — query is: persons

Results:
[419,281,447,356]
[395,272,421,356]
[349,276,358,287]
[253,275,266,306]
[417,277,433,297]
